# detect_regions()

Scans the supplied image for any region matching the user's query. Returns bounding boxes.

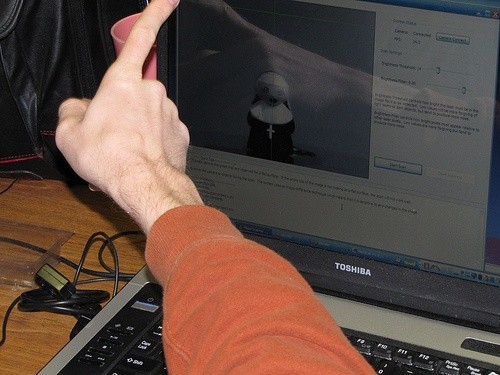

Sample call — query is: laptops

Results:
[34,0,500,375]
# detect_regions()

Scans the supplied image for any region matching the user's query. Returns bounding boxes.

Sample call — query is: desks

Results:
[0,178,149,374]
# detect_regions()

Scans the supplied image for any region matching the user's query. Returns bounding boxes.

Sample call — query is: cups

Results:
[110,12,158,80]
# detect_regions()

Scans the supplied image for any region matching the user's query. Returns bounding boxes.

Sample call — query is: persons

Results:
[199,2,499,154]
[55,1,378,375]
